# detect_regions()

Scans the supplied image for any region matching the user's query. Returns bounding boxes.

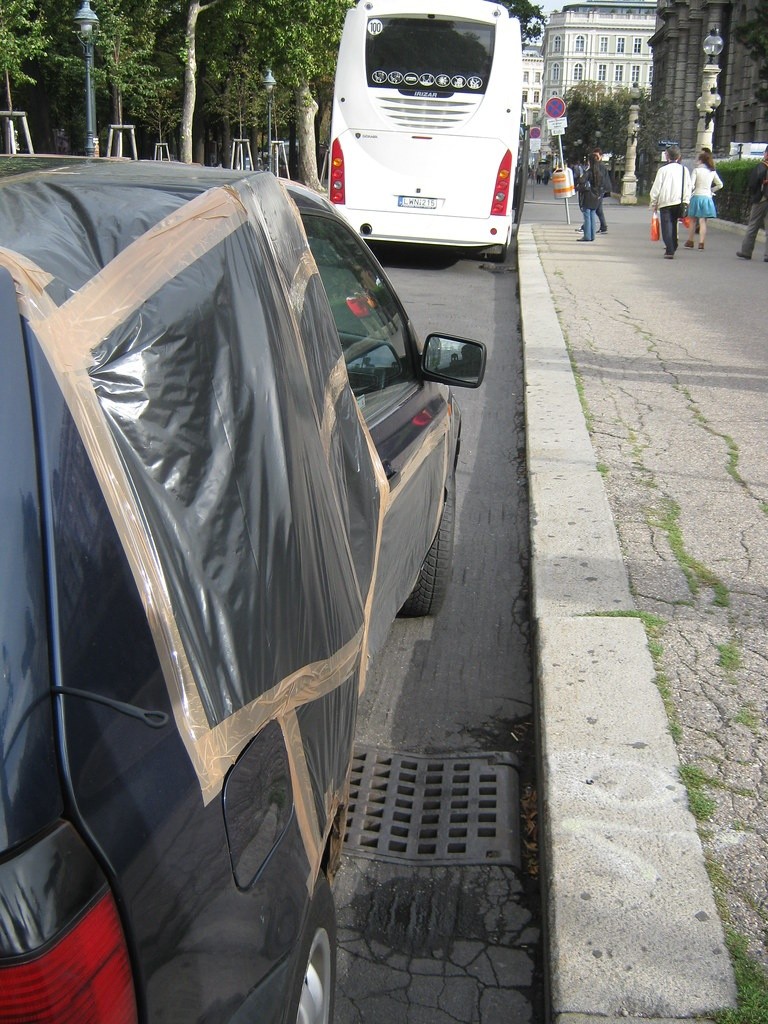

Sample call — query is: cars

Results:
[0,153,492,1024]
[238,157,263,170]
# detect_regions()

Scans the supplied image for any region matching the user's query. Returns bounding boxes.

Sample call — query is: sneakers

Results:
[575,226,584,232]
[596,226,608,234]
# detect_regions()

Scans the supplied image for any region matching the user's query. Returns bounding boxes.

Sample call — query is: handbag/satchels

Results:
[678,203,688,218]
[577,181,591,191]
[651,212,659,241]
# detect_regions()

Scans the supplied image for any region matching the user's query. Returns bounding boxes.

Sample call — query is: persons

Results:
[650,145,691,259]
[529,165,551,185]
[736,145,768,261]
[683,147,724,249]
[566,147,612,241]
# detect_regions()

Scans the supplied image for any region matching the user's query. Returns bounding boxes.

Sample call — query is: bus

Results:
[326,0,523,263]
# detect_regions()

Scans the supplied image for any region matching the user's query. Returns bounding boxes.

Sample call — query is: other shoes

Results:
[698,243,704,249]
[764,258,768,262]
[684,240,694,247]
[664,253,673,259]
[577,238,594,241]
[736,252,751,259]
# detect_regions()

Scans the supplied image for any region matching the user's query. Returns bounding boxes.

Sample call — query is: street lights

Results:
[695,27,724,161]
[261,70,277,173]
[619,83,641,206]
[72,0,100,158]
